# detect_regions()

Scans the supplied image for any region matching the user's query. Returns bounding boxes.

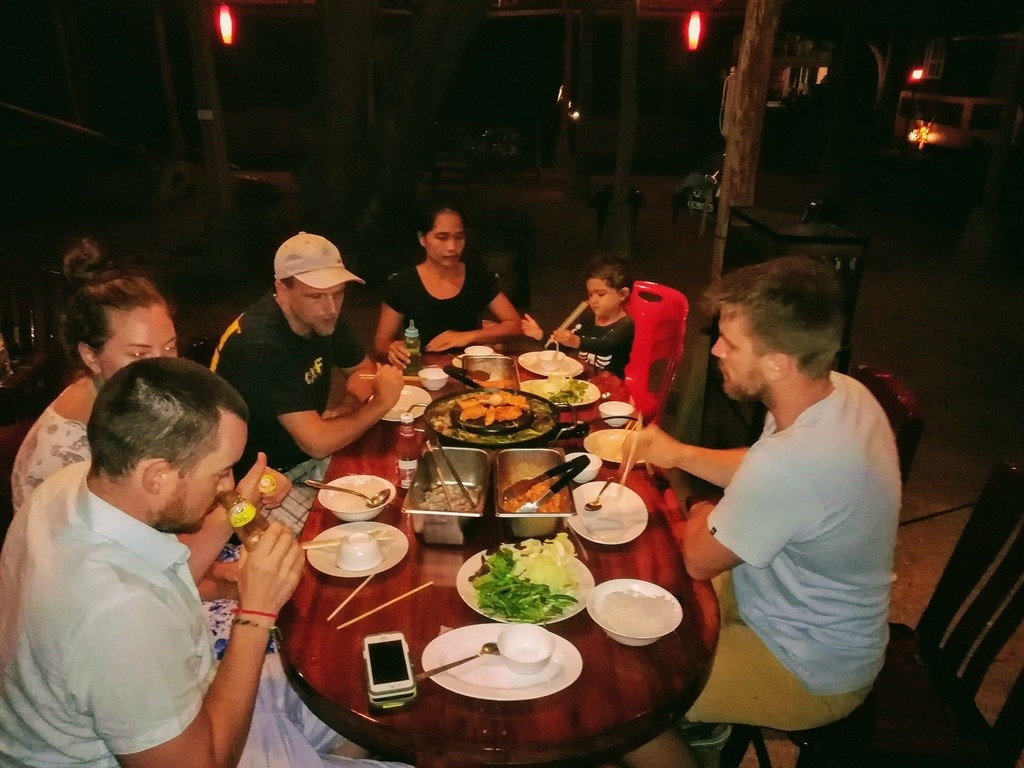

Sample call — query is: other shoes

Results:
[676,723,732,746]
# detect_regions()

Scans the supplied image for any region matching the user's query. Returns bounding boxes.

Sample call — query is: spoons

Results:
[414,642,500,682]
[585,475,615,511]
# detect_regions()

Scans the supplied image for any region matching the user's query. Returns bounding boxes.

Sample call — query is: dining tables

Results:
[274,333,722,768]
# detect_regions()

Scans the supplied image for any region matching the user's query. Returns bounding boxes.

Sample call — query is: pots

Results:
[408,388,578,455]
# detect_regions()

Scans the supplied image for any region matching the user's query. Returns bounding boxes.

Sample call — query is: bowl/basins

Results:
[318,474,396,524]
[464,346,494,357]
[417,367,450,390]
[586,578,684,647]
[335,532,385,572]
[537,350,565,371]
[598,401,635,427]
[563,452,602,483]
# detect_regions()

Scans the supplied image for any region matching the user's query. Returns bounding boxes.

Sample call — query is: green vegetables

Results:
[472,550,579,625]
[549,380,588,403]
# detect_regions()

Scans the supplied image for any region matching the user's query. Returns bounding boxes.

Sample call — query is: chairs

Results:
[714,359,1024,768]
[622,279,691,427]
[0,254,67,430]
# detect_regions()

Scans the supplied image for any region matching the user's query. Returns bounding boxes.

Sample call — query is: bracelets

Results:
[231,609,278,618]
[232,619,258,627]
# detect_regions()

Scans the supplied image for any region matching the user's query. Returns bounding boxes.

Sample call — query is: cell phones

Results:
[364,631,417,708]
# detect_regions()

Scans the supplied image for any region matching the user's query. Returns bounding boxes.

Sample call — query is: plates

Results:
[451,352,512,369]
[518,351,584,378]
[456,543,597,625]
[368,384,433,421]
[307,521,410,578]
[519,379,600,406]
[422,622,584,702]
[568,481,648,546]
[583,428,648,464]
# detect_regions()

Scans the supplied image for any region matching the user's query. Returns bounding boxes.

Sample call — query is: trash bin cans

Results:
[594,184,642,237]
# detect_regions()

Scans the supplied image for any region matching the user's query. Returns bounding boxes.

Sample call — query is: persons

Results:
[523,260,635,377]
[1,357,413,768]
[211,231,403,536]
[373,200,523,372]
[623,254,903,767]
[12,236,280,659]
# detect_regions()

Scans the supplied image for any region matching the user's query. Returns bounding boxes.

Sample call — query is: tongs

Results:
[426,433,477,512]
[443,365,490,390]
[500,455,590,513]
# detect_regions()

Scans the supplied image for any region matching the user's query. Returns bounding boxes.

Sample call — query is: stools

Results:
[590,174,648,244]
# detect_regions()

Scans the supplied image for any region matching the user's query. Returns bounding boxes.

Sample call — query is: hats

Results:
[274,231,366,289]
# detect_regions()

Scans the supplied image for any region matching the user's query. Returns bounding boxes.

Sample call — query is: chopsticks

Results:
[359,373,423,381]
[608,419,638,452]
[326,573,434,630]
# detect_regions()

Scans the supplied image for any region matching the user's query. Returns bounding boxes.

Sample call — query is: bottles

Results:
[394,413,423,496]
[219,490,270,554]
[401,319,421,376]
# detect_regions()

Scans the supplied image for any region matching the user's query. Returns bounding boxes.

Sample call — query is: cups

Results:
[497,623,556,675]
